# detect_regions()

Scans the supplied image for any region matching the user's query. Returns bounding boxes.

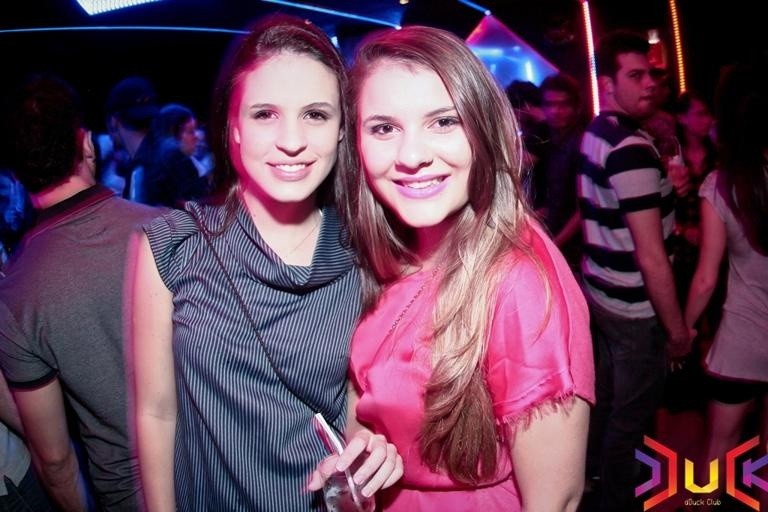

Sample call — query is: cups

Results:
[316,448,380,512]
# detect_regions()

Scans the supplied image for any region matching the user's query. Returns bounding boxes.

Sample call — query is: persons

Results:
[118,14,404,511]
[1,64,210,512]
[332,23,596,512]
[518,33,765,512]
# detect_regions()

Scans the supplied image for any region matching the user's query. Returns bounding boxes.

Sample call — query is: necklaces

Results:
[244,196,322,264]
[366,252,448,373]
